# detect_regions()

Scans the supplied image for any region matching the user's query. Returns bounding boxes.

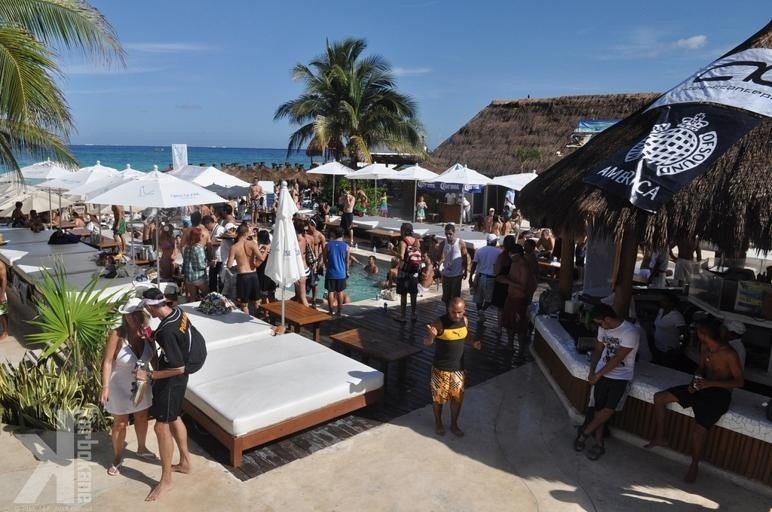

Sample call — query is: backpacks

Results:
[401,237,422,273]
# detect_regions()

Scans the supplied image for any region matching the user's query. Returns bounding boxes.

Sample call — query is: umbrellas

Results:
[0,157,231,290]
[306,159,355,206]
[387,163,439,222]
[344,161,399,201]
[422,164,493,228]
[488,170,538,191]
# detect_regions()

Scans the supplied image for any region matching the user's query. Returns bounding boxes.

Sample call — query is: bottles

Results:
[376,294,379,300]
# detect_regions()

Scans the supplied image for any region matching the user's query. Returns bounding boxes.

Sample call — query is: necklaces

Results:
[706,343,724,363]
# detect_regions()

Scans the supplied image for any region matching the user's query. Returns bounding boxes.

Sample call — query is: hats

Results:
[139,296,165,307]
[487,234,500,244]
[118,297,144,314]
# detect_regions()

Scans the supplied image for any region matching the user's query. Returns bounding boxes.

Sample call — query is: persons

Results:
[132,289,192,501]
[423,296,481,437]
[578,292,637,319]
[1,179,590,344]
[575,304,640,461]
[725,321,748,373]
[653,293,690,366]
[641,239,701,286]
[643,318,745,482]
[99,296,157,474]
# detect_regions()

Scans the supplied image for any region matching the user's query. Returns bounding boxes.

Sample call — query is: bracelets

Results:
[100,385,109,389]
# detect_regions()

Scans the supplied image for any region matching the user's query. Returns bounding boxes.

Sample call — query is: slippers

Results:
[109,463,122,476]
[135,450,158,461]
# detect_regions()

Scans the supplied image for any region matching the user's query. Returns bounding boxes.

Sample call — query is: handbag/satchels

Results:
[539,289,562,314]
[48,230,80,244]
[185,326,207,375]
[196,291,237,315]
[305,243,318,266]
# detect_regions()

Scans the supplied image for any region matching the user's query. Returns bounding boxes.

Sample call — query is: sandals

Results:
[586,440,605,460]
[574,425,589,451]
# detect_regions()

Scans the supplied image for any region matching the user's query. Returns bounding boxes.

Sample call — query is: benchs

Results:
[328,326,423,395]
[321,221,359,239]
[365,227,401,249]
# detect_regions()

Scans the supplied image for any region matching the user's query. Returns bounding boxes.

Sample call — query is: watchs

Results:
[147,371,153,380]
